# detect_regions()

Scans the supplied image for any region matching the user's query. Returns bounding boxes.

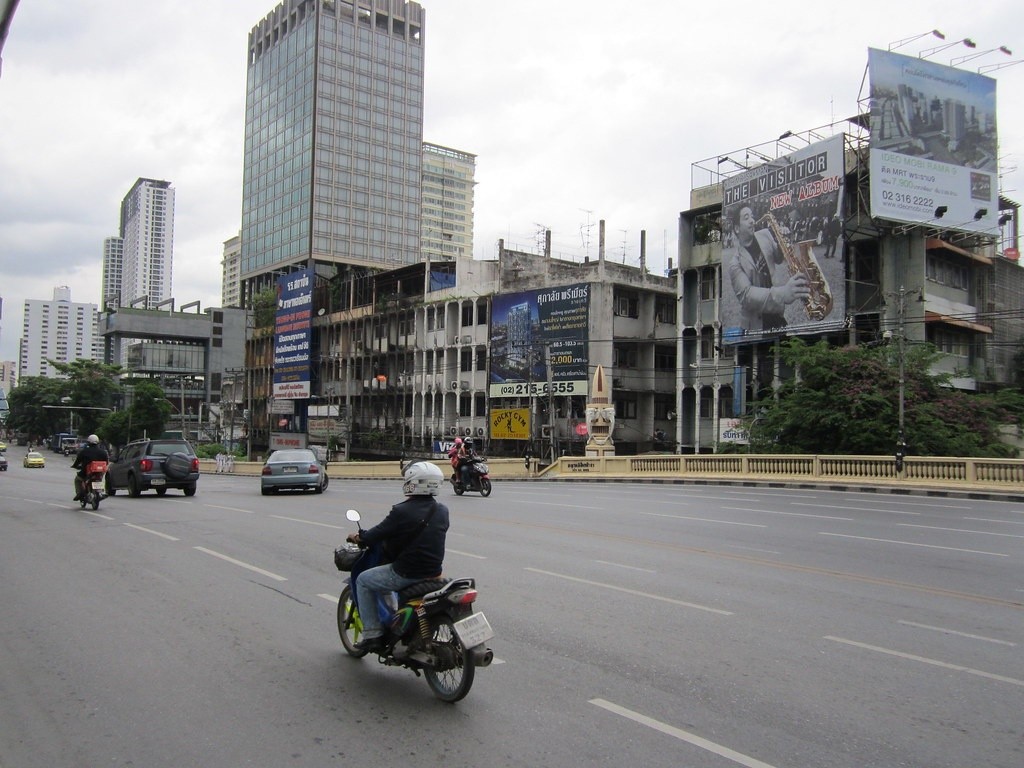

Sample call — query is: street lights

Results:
[154,398,186,439]
[883,330,904,471]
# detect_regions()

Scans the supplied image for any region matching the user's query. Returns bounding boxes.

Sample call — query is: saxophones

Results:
[751,210,835,324]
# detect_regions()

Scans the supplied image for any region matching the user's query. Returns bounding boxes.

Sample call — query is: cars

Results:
[262,449,329,496]
[78,439,87,447]
[0,453,8,471]
[0,442,7,452]
[23,452,45,467]
[105,438,200,498]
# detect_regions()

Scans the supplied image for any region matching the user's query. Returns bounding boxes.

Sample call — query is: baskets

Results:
[334,548,364,571]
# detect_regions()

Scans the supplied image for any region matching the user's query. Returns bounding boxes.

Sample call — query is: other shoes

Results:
[73,494,81,501]
[354,635,382,652]
[467,485,471,491]
[456,478,460,483]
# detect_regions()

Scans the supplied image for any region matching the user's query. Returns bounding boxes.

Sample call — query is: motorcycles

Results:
[450,457,492,497]
[334,510,494,703]
[71,457,108,510]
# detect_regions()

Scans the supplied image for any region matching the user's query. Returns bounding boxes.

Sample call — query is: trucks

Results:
[52,433,77,454]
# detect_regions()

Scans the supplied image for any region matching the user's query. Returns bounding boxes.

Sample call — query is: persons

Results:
[727,203,841,332]
[27,444,34,450]
[72,434,109,502]
[215,450,236,475]
[344,461,449,650]
[448,437,487,491]
[64,446,70,454]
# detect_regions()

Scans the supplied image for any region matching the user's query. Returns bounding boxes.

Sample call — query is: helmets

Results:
[87,434,99,444]
[404,461,444,496]
[464,437,473,444]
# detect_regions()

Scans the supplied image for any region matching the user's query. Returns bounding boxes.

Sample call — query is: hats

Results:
[454,437,462,444]
